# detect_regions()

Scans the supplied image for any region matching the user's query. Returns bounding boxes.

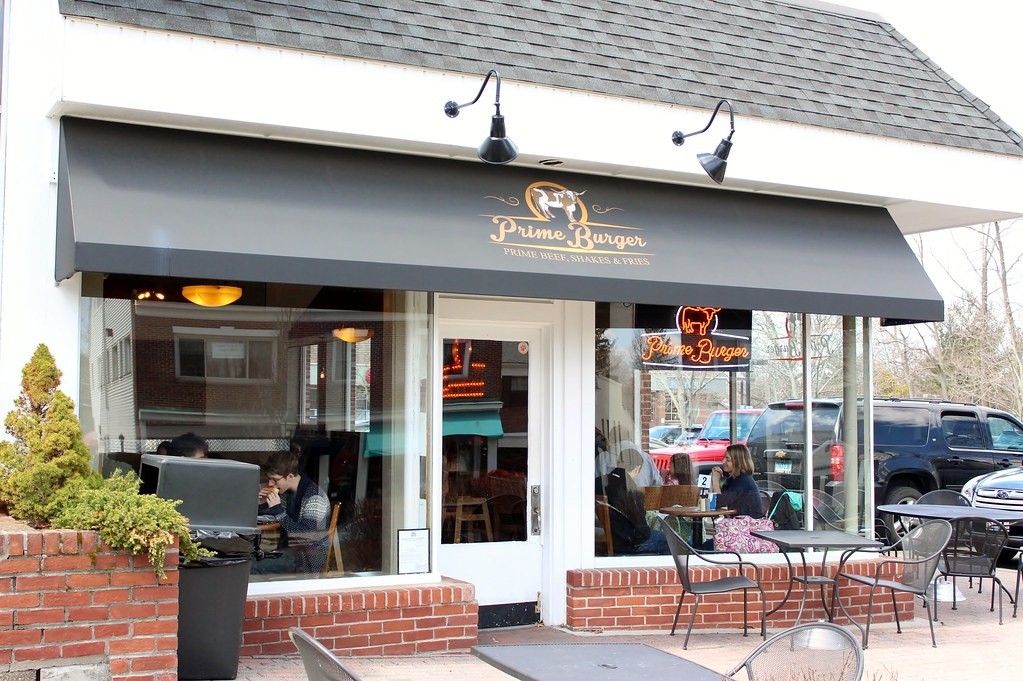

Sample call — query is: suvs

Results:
[958,467,1023,572]
[649,408,766,485]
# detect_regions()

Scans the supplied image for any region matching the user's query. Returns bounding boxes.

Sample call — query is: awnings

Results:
[443,410,504,439]
[363,426,402,457]
[54,116,944,327]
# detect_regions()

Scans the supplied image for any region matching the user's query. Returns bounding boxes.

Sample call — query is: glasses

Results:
[264,473,289,484]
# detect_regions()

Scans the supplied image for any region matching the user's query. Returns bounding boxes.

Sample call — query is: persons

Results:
[596,449,673,555]
[251,451,331,573]
[596,426,662,486]
[157,432,208,458]
[645,453,707,542]
[710,444,779,553]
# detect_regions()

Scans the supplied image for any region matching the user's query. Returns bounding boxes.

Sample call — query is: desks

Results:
[469,642,736,681]
[749,531,884,654]
[256,522,280,534]
[877,504,1023,617]
[659,507,737,554]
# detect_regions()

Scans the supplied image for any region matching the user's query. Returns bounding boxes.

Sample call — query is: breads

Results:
[266,486,279,494]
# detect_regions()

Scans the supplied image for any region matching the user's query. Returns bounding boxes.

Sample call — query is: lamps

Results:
[181,285,242,307]
[672,99,735,184]
[444,68,519,165]
[332,328,375,343]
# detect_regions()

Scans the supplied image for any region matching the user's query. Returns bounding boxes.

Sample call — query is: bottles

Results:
[699,494,710,511]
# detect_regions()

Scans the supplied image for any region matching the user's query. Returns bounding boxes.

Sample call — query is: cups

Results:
[708,493,718,510]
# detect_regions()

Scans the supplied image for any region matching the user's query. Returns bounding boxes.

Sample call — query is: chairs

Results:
[595,483,1023,681]
[320,502,342,573]
[286,625,360,681]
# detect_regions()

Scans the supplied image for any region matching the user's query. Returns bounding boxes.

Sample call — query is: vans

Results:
[745,397,1023,551]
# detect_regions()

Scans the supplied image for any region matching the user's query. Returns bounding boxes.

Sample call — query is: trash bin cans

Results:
[139,452,262,681]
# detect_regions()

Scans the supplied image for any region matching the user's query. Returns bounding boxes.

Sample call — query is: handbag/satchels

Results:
[714,514,779,553]
[636,505,694,552]
